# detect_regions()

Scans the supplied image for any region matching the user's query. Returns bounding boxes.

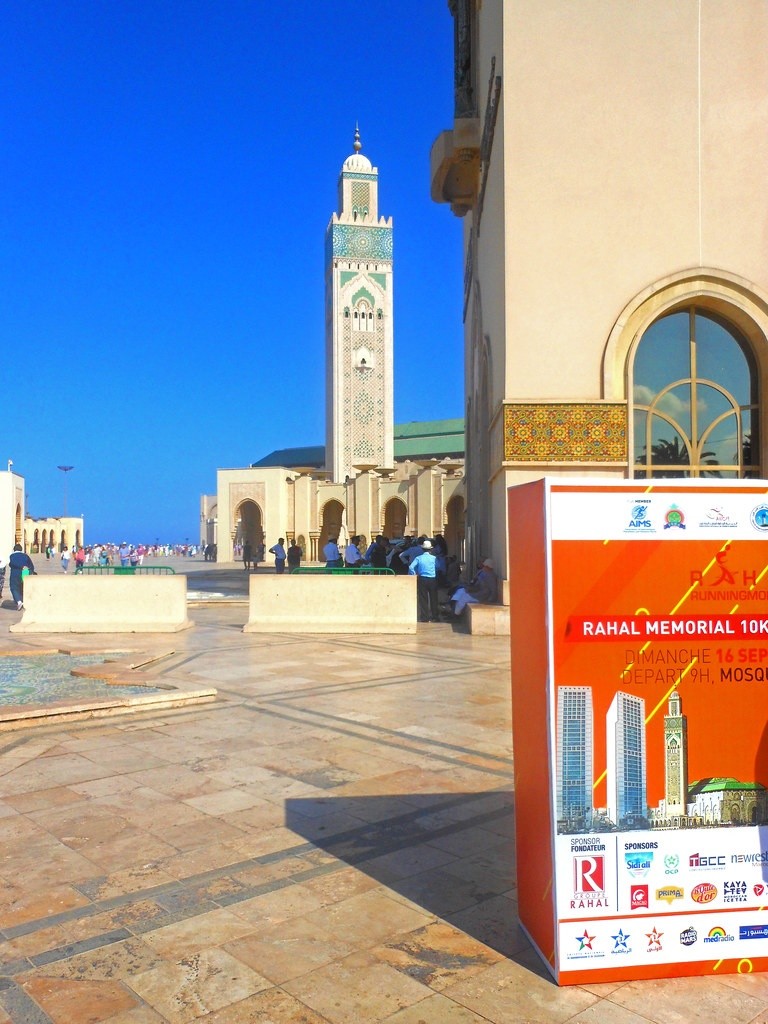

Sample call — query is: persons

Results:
[0,544,34,610]
[287,539,302,573]
[345,535,364,575]
[46,539,264,576]
[366,535,495,623]
[269,538,286,575]
[323,535,342,569]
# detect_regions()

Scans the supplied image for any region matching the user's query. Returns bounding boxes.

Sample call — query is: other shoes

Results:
[421,616,440,622]
[17,603,23,611]
[64,571,67,573]
[74,569,82,574]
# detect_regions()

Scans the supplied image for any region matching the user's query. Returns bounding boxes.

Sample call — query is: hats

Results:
[420,541,434,549]
[328,535,338,541]
[483,559,496,569]
[350,535,361,541]
